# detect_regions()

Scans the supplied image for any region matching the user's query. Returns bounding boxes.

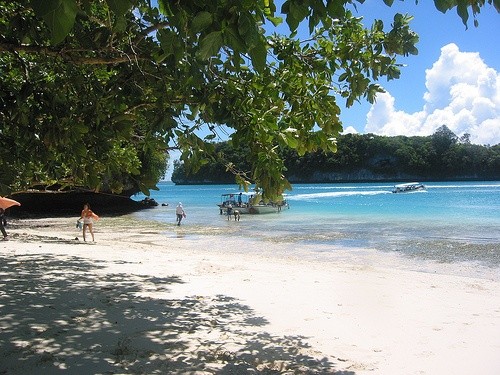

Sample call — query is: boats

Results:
[391,182,424,193]
[213,192,289,213]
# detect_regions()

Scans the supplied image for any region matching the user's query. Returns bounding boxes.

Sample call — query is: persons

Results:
[249,194,254,207]
[265,201,283,213]
[222,200,232,220]
[234,210,240,222]
[176,201,184,226]
[238,192,243,207]
[78,204,98,242]
[229,194,235,204]
[0,208,8,241]
